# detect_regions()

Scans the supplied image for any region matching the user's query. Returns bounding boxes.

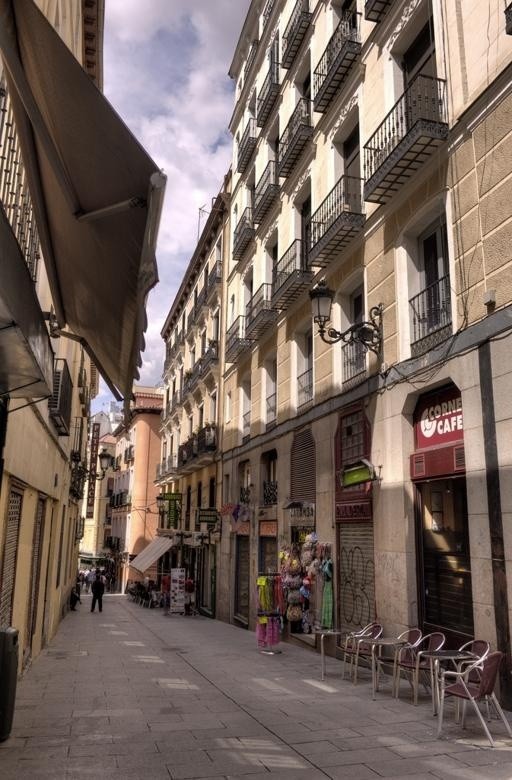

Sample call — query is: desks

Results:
[315,629,352,681]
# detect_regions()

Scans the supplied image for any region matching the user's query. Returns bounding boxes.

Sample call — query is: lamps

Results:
[70,447,114,485]
[309,280,384,364]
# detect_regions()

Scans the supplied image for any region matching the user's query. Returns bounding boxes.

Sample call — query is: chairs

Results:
[128,587,152,609]
[341,622,512,749]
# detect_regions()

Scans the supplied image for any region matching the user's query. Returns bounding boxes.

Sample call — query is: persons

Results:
[71,587,82,611]
[89,574,105,613]
[78,567,116,593]
[128,575,168,607]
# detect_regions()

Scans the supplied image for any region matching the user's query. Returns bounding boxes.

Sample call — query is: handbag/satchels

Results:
[286,557,303,621]
